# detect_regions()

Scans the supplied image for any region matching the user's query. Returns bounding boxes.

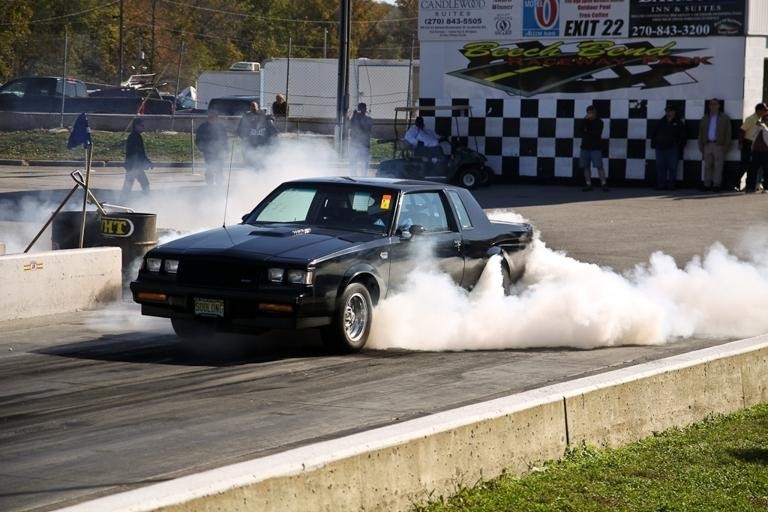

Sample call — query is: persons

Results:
[577,105,610,193]
[233,101,280,175]
[195,106,229,186]
[650,105,686,191]
[122,117,153,191]
[273,94,290,134]
[347,102,373,178]
[726,102,768,192]
[373,197,414,228]
[404,116,445,173]
[697,98,732,193]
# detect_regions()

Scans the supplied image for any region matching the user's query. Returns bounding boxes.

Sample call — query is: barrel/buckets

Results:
[100,211,157,289]
[52,211,100,250]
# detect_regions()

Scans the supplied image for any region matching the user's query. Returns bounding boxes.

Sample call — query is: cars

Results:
[0,74,194,114]
[130,172,538,358]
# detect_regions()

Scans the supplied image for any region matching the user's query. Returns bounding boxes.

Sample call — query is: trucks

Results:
[193,55,418,119]
